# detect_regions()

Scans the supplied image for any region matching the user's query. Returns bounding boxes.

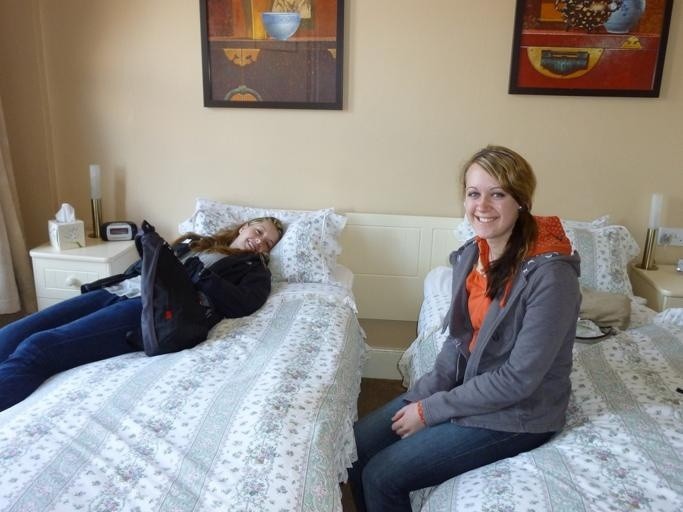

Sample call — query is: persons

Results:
[0,215,284,416]
[346,146,582,512]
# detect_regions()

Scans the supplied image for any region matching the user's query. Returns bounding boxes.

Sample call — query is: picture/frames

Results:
[507,1,674,100]
[198,2,346,112]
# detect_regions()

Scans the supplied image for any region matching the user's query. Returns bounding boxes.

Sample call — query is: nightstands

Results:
[28,232,139,315]
[629,260,682,315]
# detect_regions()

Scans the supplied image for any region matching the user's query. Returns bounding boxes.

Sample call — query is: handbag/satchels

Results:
[575,285,631,340]
[134,221,209,356]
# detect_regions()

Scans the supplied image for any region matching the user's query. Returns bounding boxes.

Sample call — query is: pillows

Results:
[465,212,640,322]
[189,202,335,283]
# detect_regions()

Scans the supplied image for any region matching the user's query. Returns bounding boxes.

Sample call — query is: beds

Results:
[0,262,361,512]
[421,273,683,512]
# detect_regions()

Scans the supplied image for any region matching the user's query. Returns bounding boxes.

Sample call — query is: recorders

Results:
[100,221,138,241]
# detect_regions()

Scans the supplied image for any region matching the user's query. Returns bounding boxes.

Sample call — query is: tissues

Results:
[48,203,86,251]
